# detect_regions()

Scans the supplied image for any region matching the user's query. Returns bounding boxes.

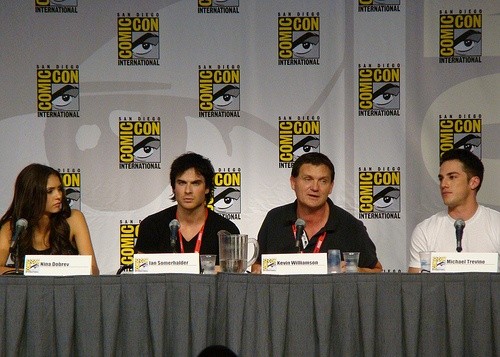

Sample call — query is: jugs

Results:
[217,230,259,275]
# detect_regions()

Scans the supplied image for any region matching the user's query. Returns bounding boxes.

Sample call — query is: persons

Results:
[0,163,99,275]
[134,152,240,272]
[408,149,500,273]
[250,152,383,273]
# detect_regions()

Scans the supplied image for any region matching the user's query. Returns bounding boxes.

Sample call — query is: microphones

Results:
[169,219,181,247]
[295,219,306,248]
[454,218,466,252]
[9,218,28,253]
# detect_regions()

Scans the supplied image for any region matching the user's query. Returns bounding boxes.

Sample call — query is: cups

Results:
[343,252,360,273]
[327,248,341,274]
[420,251,434,273]
[200,255,217,275]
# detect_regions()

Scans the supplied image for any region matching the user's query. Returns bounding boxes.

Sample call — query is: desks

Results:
[0,273,500,357]
